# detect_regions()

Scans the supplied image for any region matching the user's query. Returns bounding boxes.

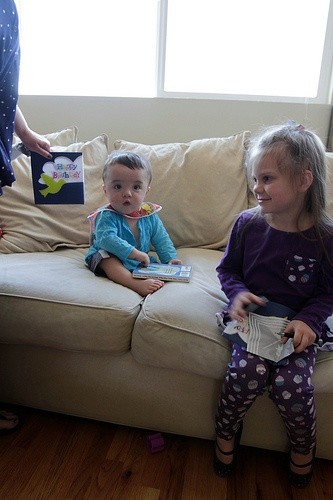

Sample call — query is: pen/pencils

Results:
[274,331,295,338]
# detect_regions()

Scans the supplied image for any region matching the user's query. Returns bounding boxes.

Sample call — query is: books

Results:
[131,263,194,283]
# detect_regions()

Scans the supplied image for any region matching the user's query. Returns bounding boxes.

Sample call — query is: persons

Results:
[84,151,182,297]
[0,0,53,202]
[214,123,333,415]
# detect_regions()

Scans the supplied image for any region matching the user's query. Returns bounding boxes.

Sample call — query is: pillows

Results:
[0,128,111,255]
[114,131,245,250]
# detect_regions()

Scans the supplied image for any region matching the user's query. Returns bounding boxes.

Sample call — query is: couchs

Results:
[1,127,333,463]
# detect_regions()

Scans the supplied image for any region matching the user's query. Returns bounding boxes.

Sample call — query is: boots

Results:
[212,419,244,477]
[288,436,317,488]
